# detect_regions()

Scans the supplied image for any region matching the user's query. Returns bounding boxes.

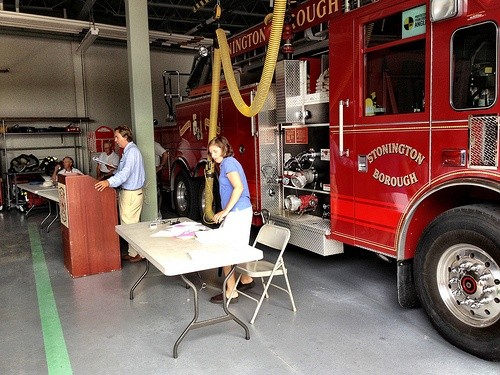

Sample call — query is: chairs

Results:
[225,224,297,324]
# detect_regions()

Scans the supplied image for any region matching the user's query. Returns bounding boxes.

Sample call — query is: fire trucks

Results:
[162,0,500,362]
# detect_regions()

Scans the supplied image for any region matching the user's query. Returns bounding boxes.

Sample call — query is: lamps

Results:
[72,0,101,56]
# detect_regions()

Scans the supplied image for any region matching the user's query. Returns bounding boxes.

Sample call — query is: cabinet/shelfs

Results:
[0,119,84,211]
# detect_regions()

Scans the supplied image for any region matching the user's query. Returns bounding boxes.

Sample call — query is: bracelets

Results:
[103,174,105,177]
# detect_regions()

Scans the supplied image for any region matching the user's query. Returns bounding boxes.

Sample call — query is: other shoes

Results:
[238,280,255,291]
[210,293,240,304]
[121,255,144,262]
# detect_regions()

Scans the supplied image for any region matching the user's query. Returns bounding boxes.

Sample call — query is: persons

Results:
[97,141,119,180]
[209,136,254,304]
[52,157,84,181]
[95,124,146,262]
[154,141,168,219]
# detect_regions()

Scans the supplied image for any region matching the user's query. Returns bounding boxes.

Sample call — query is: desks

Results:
[17,182,60,234]
[114,217,264,360]
